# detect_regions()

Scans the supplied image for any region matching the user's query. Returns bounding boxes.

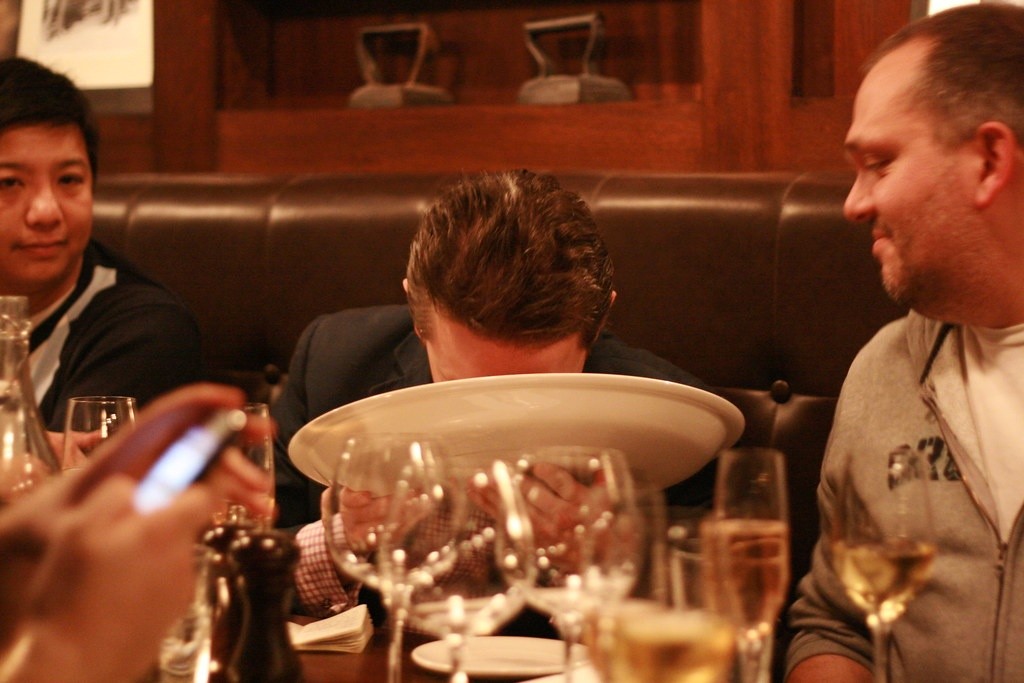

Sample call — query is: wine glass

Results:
[826,446,937,683]
[326,433,465,683]
[495,447,644,683]
[707,449,790,683]
[378,458,537,683]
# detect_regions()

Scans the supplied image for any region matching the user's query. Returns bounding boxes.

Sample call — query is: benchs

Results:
[79,170,910,601]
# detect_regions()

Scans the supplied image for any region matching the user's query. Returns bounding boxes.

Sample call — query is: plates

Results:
[288,373,744,490]
[520,672,596,683]
[411,636,592,677]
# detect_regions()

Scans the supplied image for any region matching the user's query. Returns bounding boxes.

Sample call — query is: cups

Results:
[0,315,33,404]
[663,521,704,613]
[62,397,135,477]
[0,395,24,505]
[577,505,744,683]
[158,546,212,683]
[212,404,276,537]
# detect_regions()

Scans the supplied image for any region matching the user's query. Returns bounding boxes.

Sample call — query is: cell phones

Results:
[134,407,248,512]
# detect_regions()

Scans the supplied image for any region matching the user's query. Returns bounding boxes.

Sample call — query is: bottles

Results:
[0,294,61,477]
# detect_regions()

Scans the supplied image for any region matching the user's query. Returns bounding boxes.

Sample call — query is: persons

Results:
[0,54,199,465]
[786,5,1024,683]
[245,169,742,613]
[0,380,278,683]
